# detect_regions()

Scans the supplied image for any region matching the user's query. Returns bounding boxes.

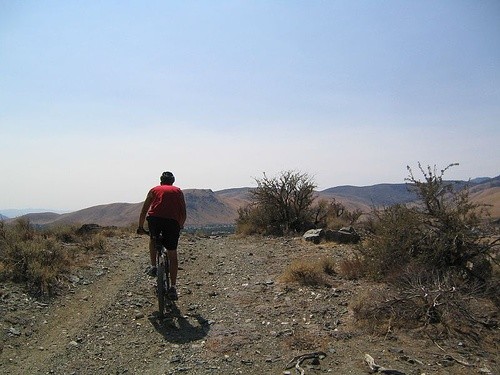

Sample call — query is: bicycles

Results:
[138,229,177,323]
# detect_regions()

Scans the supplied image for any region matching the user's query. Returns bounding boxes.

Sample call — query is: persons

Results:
[137,171,187,301]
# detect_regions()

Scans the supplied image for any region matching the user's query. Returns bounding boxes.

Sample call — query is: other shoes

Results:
[168,287,179,301]
[147,268,157,277]
